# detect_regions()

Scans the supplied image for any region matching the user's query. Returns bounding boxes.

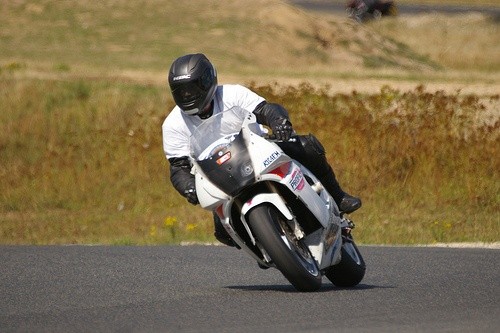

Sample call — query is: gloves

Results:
[272,117,294,141]
[184,180,201,205]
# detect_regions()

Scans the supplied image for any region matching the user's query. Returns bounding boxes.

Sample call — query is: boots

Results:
[316,165,363,213]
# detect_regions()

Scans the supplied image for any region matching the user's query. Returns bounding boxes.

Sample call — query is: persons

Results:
[161,52,363,269]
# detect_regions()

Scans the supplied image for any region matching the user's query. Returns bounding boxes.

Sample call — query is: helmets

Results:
[168,53,218,116]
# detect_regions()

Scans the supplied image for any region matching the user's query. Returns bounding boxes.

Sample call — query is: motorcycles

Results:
[182,106,367,290]
